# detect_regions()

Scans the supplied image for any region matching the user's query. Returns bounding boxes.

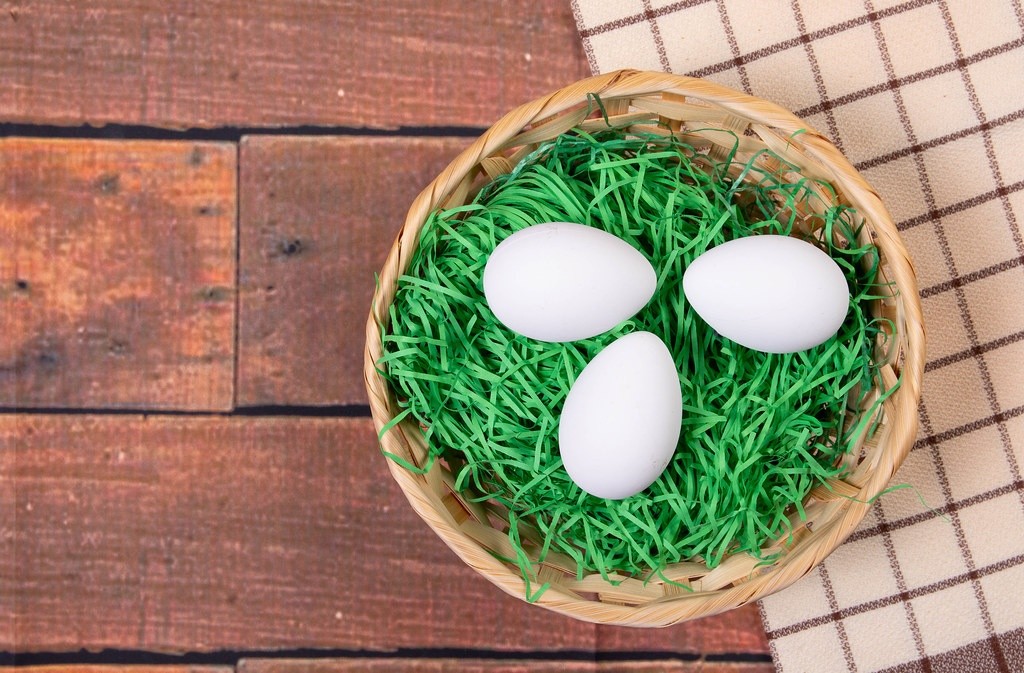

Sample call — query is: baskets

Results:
[366,69,925,627]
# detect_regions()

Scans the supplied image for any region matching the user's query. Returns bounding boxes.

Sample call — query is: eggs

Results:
[558,331,683,500]
[681,235,850,353]
[482,222,658,342]
